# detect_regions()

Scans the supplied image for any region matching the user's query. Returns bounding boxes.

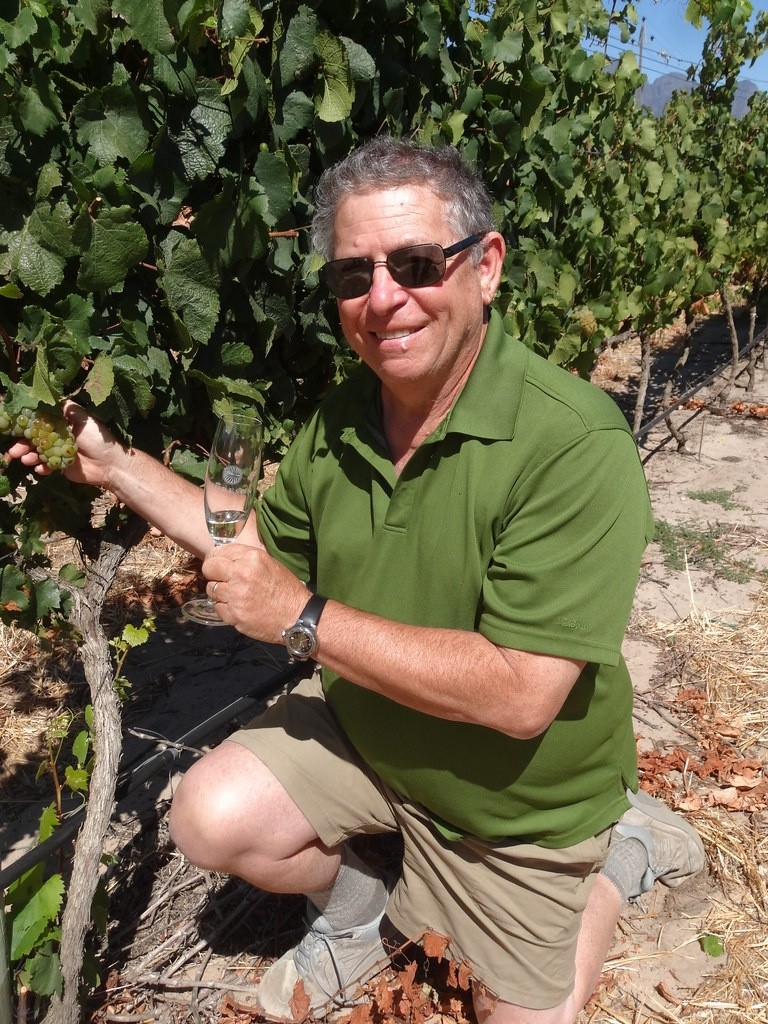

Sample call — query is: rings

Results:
[212,581,224,603]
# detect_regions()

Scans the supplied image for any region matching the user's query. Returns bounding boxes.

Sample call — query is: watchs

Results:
[282,593,330,661]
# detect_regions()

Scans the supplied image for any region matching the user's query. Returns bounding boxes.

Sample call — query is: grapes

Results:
[0,401,78,471]
[651,295,660,315]
[572,305,597,337]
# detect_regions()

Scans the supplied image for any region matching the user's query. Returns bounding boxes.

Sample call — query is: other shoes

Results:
[612,787,704,903]
[256,872,404,1021]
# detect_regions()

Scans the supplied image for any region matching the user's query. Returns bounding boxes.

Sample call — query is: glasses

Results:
[318,230,490,299]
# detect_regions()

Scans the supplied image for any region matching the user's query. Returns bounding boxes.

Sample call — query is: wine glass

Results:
[181,412,262,626]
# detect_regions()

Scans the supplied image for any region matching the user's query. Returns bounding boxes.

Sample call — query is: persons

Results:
[9,132,708,1024]
